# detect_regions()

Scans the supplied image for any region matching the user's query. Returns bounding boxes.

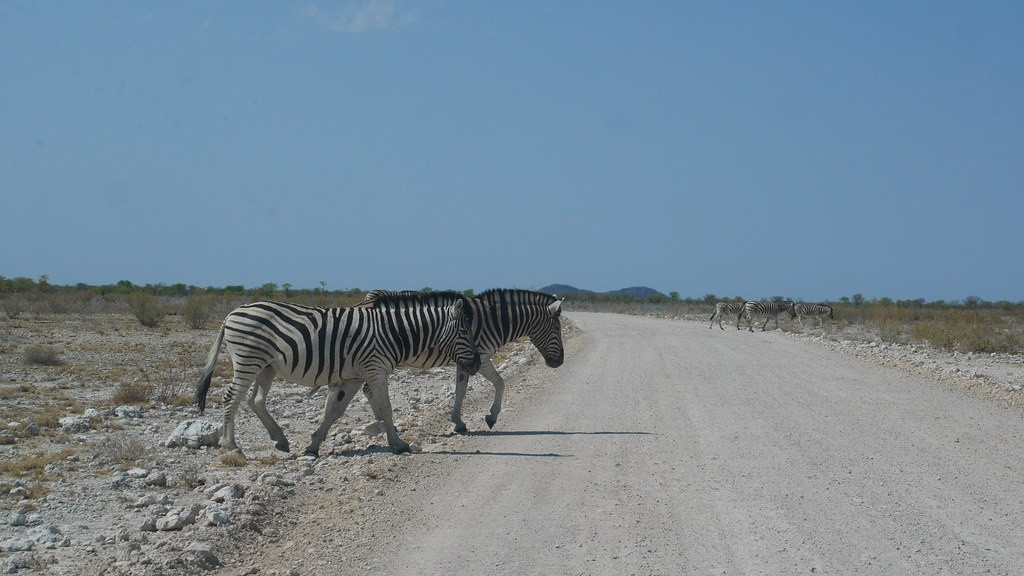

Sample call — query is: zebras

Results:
[191,288,565,454]
[709,301,833,332]
